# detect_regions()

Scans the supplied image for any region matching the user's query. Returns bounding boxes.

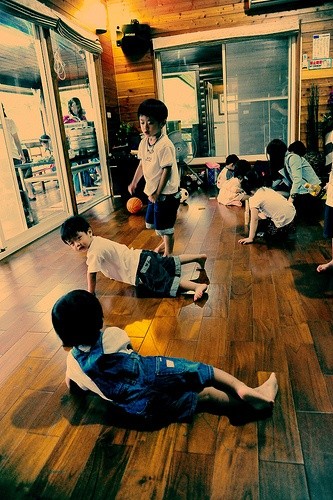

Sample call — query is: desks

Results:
[15,161,52,191]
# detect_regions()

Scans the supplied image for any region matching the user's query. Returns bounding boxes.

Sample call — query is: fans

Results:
[168,130,198,167]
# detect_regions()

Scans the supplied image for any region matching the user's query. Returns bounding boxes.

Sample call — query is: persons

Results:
[2,103,31,223]
[50,289,277,424]
[317,131,333,272]
[39,135,56,173]
[60,215,207,301]
[62,97,94,188]
[215,139,322,245]
[19,146,35,201]
[128,98,181,257]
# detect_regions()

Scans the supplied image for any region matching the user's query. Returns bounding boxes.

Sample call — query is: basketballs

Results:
[127,198,143,214]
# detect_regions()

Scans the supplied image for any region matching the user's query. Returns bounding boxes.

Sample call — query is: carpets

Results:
[51,193,93,208]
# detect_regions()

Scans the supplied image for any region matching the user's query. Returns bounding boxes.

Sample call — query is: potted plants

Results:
[306,152,329,187]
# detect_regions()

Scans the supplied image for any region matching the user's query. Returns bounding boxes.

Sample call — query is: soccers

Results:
[179,187,190,203]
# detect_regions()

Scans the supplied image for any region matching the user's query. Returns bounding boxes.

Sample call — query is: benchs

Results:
[26,172,58,201]
